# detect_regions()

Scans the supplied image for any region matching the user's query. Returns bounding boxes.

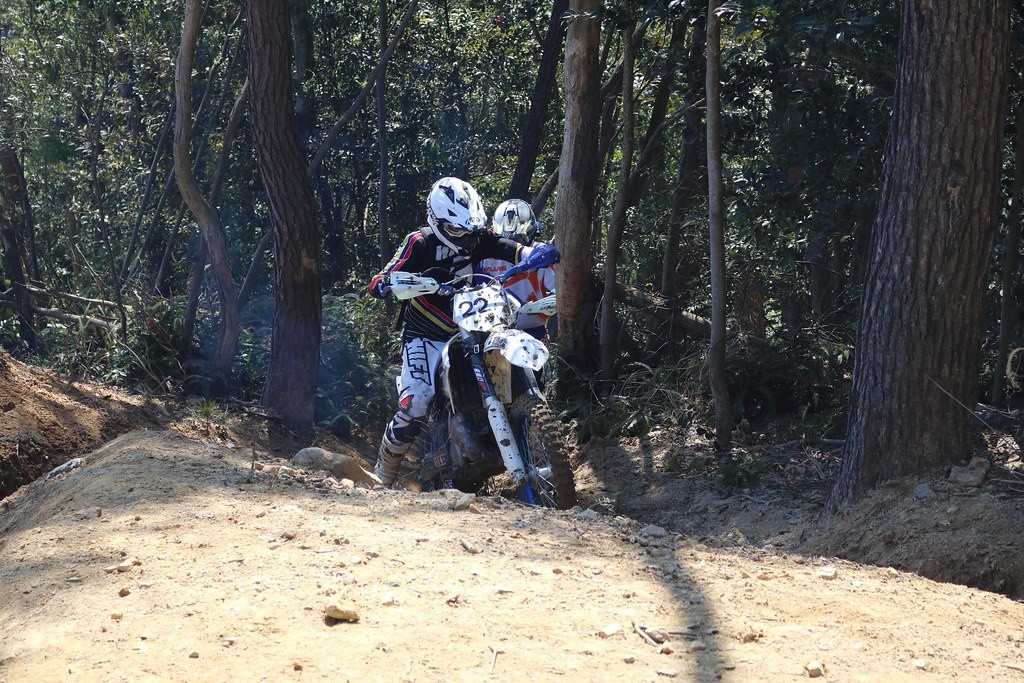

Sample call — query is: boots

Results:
[373,423,413,489]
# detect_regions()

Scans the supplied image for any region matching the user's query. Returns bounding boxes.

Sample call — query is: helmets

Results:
[492,199,538,247]
[426,176,488,260]
[536,221,542,233]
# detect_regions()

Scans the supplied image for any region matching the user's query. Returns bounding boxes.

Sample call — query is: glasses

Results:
[536,232,541,237]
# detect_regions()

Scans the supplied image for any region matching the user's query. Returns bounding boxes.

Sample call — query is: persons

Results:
[475,199,556,389]
[370,177,554,492]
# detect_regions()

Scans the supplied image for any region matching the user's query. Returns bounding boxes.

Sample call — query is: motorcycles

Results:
[377,245,578,510]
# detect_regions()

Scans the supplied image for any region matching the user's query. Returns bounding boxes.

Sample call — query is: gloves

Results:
[377,275,392,298]
